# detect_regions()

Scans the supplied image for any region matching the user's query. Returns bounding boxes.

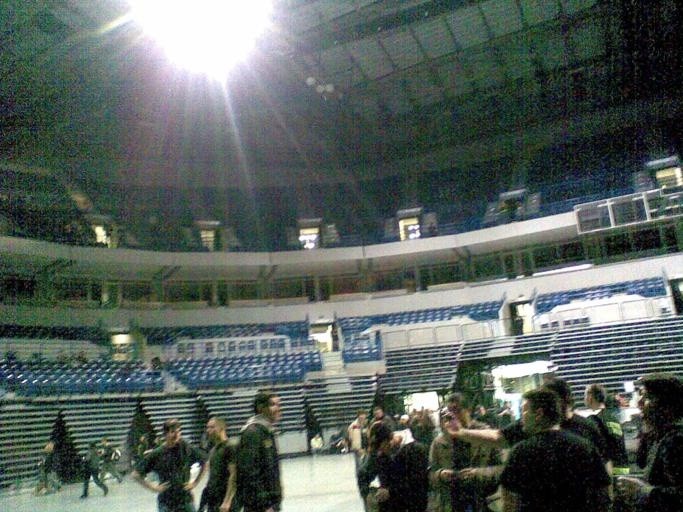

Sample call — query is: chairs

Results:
[0,358,164,393]
[336,291,502,362]
[166,310,321,387]
[534,276,664,326]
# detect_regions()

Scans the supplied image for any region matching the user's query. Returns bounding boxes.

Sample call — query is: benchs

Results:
[379,316,683,398]
[0,374,378,490]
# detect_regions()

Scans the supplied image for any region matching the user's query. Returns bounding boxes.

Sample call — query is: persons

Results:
[4,348,162,369]
[43,444,61,494]
[137,390,283,510]
[80,437,123,499]
[311,432,324,454]
[347,372,682,512]
